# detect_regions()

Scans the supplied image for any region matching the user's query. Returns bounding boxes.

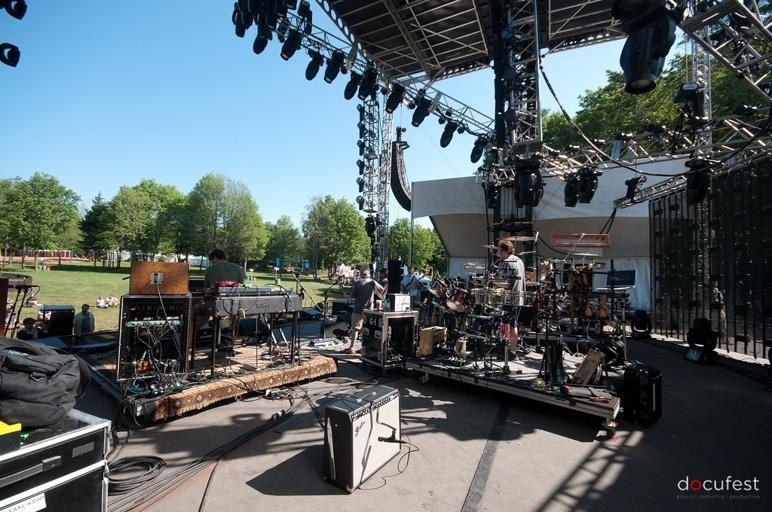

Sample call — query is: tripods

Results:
[455,271,570,378]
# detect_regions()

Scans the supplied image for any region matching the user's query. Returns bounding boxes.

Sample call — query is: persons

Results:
[16,316,50,341]
[106,294,121,307]
[36,303,52,320]
[377,271,389,301]
[710,280,734,345]
[294,269,301,279]
[96,296,108,308]
[197,248,247,329]
[496,239,528,361]
[339,265,388,354]
[414,266,432,283]
[72,303,95,335]
[400,265,421,304]
[335,262,374,287]
[5,298,24,330]
[25,293,43,308]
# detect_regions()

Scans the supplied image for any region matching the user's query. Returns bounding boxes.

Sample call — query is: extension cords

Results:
[265,387,280,397]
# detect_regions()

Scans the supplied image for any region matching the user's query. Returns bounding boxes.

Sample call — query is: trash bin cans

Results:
[38,265,46,271]
[275,278,281,285]
[249,276,253,281]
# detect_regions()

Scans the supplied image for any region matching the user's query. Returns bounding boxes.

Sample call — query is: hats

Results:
[379,268,388,275]
[360,265,371,277]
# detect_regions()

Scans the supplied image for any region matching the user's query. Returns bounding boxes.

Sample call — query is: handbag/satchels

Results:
[0,338,91,429]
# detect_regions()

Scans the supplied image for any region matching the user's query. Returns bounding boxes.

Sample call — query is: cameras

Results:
[35,321,43,326]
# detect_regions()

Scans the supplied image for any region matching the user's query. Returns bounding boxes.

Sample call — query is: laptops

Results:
[596,269,637,291]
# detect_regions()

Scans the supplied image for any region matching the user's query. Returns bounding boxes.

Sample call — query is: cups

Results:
[376,299,382,312]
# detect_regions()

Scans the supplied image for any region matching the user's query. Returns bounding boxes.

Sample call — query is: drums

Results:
[455,288,467,302]
[498,282,511,290]
[429,279,447,296]
[522,282,540,307]
[472,286,523,306]
[470,303,504,339]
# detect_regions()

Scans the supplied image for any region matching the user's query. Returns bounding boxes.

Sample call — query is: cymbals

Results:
[513,251,536,256]
[504,236,534,242]
[571,252,600,256]
[481,244,497,250]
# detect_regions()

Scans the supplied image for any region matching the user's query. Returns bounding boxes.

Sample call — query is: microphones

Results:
[377,436,407,444]
[339,275,345,278]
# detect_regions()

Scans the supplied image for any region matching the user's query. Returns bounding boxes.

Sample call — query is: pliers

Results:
[588,396,610,403]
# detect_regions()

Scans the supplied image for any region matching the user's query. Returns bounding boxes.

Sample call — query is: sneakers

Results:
[497,351,517,361]
[345,347,354,354]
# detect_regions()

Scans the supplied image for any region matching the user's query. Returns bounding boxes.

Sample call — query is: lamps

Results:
[231,0,712,214]
[629,306,718,353]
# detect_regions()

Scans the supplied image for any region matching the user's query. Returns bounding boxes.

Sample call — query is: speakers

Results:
[419,325,448,357]
[320,321,349,338]
[299,306,322,321]
[324,384,402,493]
[130,261,189,295]
[391,141,411,212]
[237,318,269,337]
